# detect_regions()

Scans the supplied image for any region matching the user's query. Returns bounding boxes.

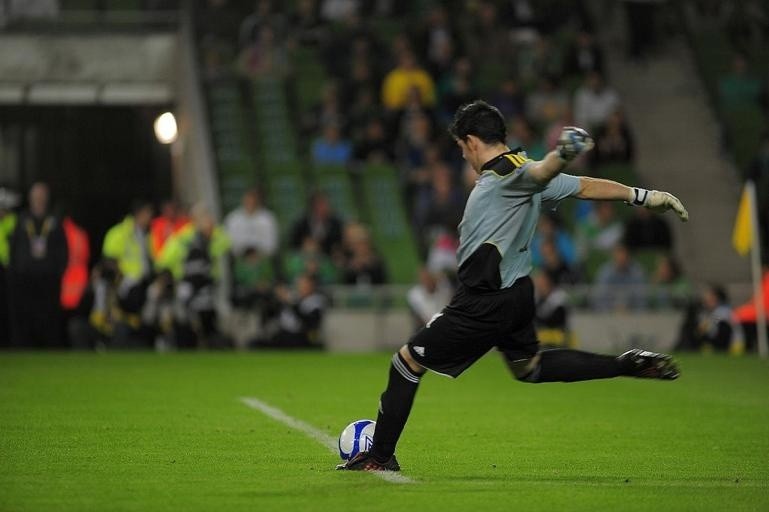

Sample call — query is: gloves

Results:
[556,126,594,161]
[624,187,690,223]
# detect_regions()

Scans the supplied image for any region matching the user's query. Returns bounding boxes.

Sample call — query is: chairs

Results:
[192,0,769,311]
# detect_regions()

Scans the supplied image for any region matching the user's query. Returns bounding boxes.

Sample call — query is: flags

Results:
[728,181,760,259]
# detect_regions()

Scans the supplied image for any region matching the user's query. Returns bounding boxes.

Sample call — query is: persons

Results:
[531,275,570,344]
[719,0,769,187]
[693,281,745,356]
[335,96,693,474]
[238,1,392,163]
[367,3,689,309]
[226,183,389,354]
[408,272,443,329]
[2,181,223,350]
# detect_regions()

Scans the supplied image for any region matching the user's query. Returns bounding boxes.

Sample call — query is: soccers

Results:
[339,418,377,464]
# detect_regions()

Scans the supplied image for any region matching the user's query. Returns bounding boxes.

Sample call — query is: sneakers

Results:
[335,451,400,471]
[617,349,680,380]
